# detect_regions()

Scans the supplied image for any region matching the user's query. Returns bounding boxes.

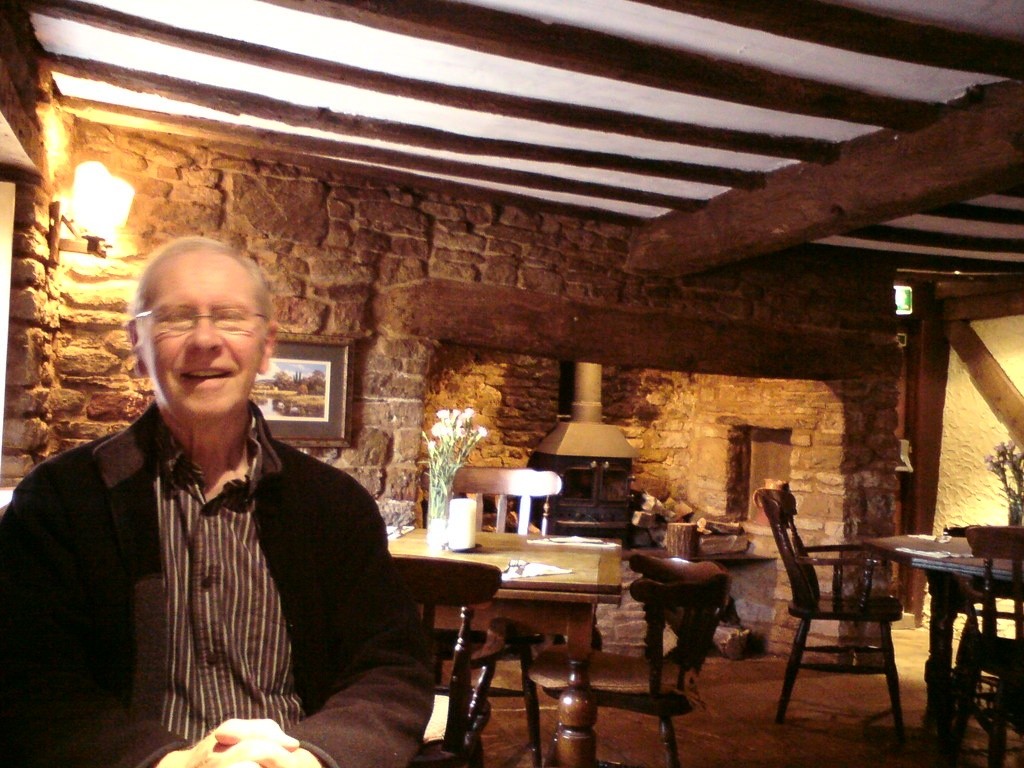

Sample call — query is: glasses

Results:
[136,308,270,341]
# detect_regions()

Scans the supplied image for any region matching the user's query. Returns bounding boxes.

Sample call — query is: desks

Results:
[866,535,1024,747]
[385,530,624,768]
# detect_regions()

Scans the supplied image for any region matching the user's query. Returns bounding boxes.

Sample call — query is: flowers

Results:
[419,407,488,517]
[985,441,1024,499]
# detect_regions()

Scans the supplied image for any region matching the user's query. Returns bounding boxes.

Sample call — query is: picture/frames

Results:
[250,332,356,449]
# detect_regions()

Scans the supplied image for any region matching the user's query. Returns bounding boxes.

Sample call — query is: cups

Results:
[447,498,477,550]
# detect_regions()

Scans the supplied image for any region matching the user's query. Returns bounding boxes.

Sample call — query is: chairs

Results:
[418,467,564,768]
[530,555,733,768]
[932,525,1024,768]
[751,487,908,746]
[389,555,517,768]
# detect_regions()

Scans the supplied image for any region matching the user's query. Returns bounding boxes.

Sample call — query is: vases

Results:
[427,517,449,559]
[1007,497,1024,526]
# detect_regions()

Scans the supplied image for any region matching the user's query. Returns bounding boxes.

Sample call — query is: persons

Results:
[0,235,436,768]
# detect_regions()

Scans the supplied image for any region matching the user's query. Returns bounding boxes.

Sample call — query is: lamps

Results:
[57,158,136,258]
